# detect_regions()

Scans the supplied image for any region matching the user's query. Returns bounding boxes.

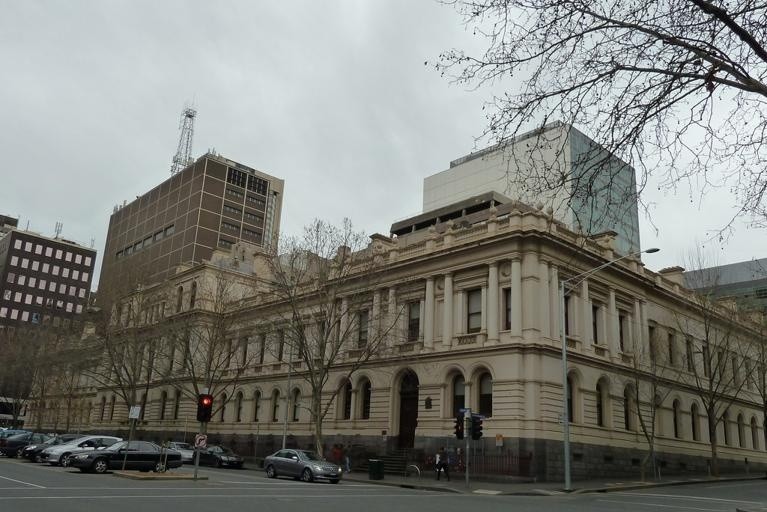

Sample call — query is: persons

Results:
[328,443,352,474]
[435,447,451,481]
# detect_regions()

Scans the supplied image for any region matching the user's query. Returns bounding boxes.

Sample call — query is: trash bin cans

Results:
[368,460,385,479]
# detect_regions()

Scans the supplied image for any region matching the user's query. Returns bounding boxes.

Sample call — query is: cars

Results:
[192,444,243,468]
[40,435,123,467]
[74,440,183,474]
[163,441,194,463]
[0,427,52,458]
[21,433,85,463]
[263,448,342,483]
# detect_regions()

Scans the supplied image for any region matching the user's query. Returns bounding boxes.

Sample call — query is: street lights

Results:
[560,248,660,491]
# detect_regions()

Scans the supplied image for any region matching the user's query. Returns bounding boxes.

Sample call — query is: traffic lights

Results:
[472,416,482,439]
[197,394,213,422]
[453,417,463,439]
